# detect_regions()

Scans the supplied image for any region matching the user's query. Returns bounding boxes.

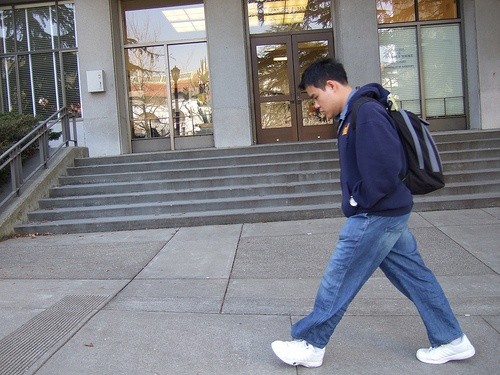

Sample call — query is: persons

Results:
[271,57,475,368]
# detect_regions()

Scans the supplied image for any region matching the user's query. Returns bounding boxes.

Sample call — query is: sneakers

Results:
[271,338,325,368]
[416,333,475,365]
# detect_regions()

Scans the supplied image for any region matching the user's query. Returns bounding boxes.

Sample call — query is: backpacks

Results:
[350,95,444,195]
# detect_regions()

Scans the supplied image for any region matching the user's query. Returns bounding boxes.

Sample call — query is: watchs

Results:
[349,196,358,207]
